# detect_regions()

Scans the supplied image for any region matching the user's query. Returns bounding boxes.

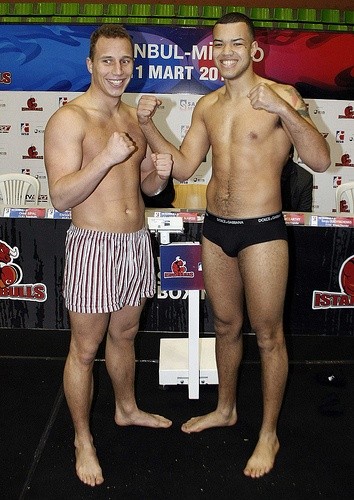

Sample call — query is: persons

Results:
[140,176,175,208]
[44,26,172,486]
[280,147,313,212]
[137,10,330,477]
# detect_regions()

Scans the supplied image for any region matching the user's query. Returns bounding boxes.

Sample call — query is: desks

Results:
[0,216,354,364]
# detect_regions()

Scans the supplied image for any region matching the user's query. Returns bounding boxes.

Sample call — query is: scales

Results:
[148,217,218,398]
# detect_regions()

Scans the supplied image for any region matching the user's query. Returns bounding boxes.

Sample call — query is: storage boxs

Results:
[284,213,304,226]
[162,213,198,221]
[309,216,354,228]
[4,208,46,218]
[48,209,71,219]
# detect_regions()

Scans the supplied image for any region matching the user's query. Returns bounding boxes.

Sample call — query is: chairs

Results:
[336,181,354,214]
[0,173,40,206]
[170,184,208,209]
